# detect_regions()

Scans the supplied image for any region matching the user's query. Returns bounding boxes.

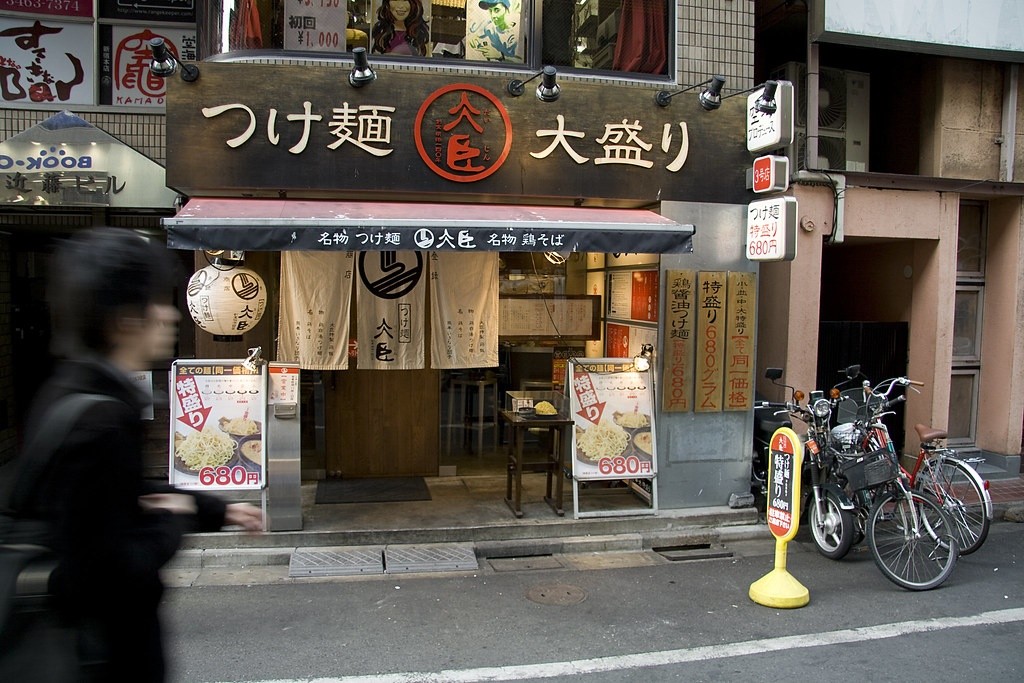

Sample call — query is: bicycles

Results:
[829,374,996,596]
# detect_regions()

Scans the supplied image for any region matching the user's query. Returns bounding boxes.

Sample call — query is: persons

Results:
[0,229,272,683]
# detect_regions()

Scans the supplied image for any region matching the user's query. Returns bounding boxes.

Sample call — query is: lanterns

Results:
[187,259,268,341]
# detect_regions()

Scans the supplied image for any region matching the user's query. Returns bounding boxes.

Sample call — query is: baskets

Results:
[836,387,888,424]
[841,447,898,491]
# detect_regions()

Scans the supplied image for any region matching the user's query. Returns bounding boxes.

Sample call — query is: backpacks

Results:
[0,394,142,683]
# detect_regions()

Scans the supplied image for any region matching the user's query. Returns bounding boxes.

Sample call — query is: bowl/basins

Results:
[576,413,652,467]
[173,436,237,475]
[230,420,262,439]
[238,434,261,481]
[519,408,536,419]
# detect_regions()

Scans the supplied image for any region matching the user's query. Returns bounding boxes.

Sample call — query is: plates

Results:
[536,414,559,419]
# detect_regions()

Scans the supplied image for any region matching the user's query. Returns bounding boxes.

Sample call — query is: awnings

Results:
[159,197,696,256]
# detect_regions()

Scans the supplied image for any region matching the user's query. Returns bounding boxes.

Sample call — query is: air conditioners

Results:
[769,62,870,175]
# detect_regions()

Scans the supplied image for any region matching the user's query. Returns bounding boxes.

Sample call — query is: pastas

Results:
[222,417,260,435]
[532,401,557,414]
[174,424,239,471]
[577,418,631,462]
[616,410,649,429]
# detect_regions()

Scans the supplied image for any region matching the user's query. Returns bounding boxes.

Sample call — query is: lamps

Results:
[509,65,561,102]
[655,75,727,112]
[721,80,778,116]
[148,37,199,83]
[348,47,376,89]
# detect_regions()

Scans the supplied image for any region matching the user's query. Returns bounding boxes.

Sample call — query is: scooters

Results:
[753,361,862,561]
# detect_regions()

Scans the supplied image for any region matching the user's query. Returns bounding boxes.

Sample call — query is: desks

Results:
[499,408,576,518]
[446,375,499,458]
